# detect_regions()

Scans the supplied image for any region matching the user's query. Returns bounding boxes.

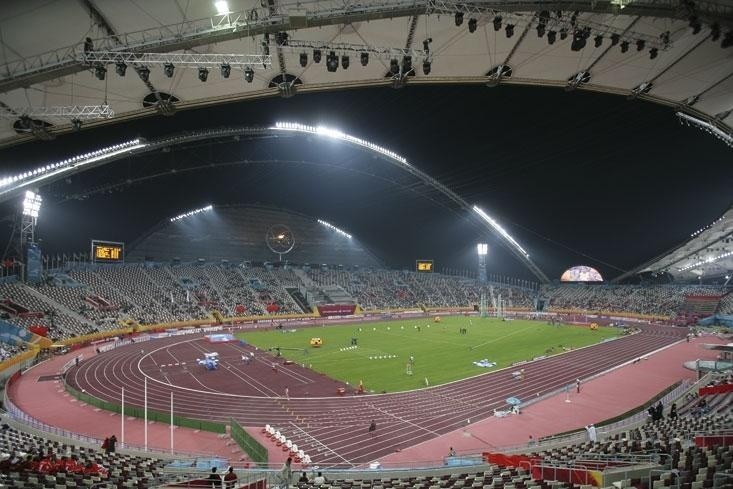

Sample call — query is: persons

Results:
[1,266,732,489]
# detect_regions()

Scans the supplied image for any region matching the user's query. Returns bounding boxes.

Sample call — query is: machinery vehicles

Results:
[309,337,322,348]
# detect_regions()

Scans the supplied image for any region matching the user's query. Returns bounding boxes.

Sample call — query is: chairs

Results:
[287,463,581,488]
[1,398,203,488]
[520,398,732,488]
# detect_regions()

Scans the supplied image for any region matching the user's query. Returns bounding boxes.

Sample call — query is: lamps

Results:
[452,9,672,60]
[11,115,86,135]
[687,17,728,50]
[261,32,433,83]
[94,58,254,85]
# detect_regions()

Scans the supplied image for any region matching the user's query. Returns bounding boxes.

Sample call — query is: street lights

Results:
[476,243,489,285]
[3,190,44,260]
